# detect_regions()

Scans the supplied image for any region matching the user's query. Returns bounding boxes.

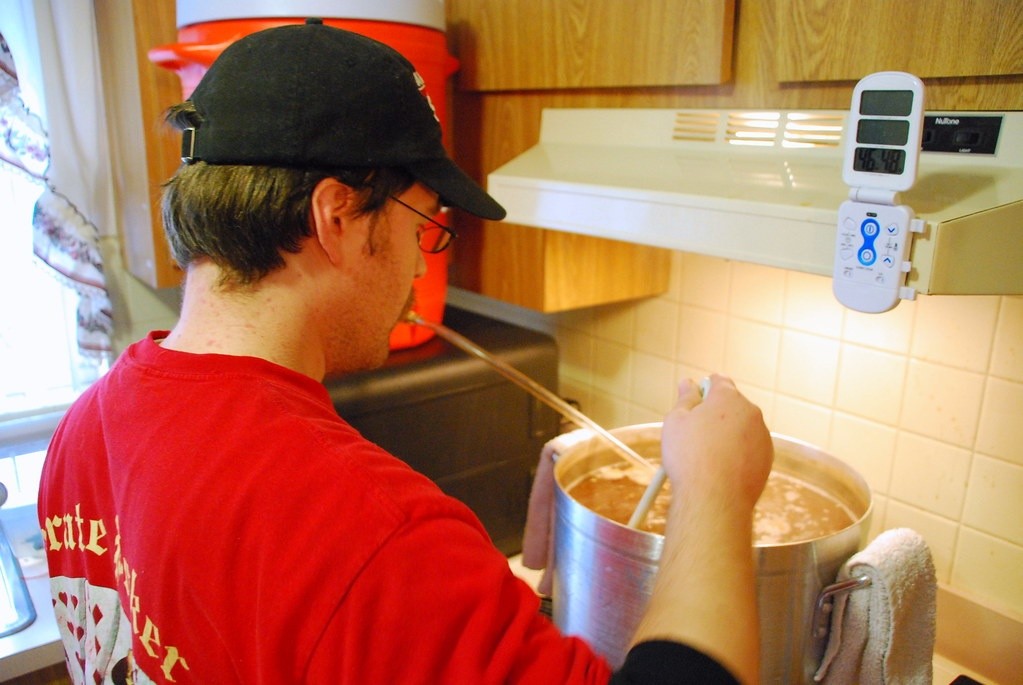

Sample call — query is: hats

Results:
[180,19,507,220]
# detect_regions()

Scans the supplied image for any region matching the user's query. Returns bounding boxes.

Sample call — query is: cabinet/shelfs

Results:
[448,0,1023,112]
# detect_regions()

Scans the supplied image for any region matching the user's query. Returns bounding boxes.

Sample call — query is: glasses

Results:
[351,175,459,253]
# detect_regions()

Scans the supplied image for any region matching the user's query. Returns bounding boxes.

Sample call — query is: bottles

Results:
[0,482,38,639]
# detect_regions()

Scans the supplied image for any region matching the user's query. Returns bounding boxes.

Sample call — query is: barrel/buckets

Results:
[145,0,459,353]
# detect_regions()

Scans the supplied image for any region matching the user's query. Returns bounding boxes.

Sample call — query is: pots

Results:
[552,420,875,685]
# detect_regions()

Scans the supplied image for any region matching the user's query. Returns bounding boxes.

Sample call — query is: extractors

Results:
[486,71,1023,315]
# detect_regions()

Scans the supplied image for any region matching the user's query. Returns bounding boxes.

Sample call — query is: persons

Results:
[36,20,774,684]
